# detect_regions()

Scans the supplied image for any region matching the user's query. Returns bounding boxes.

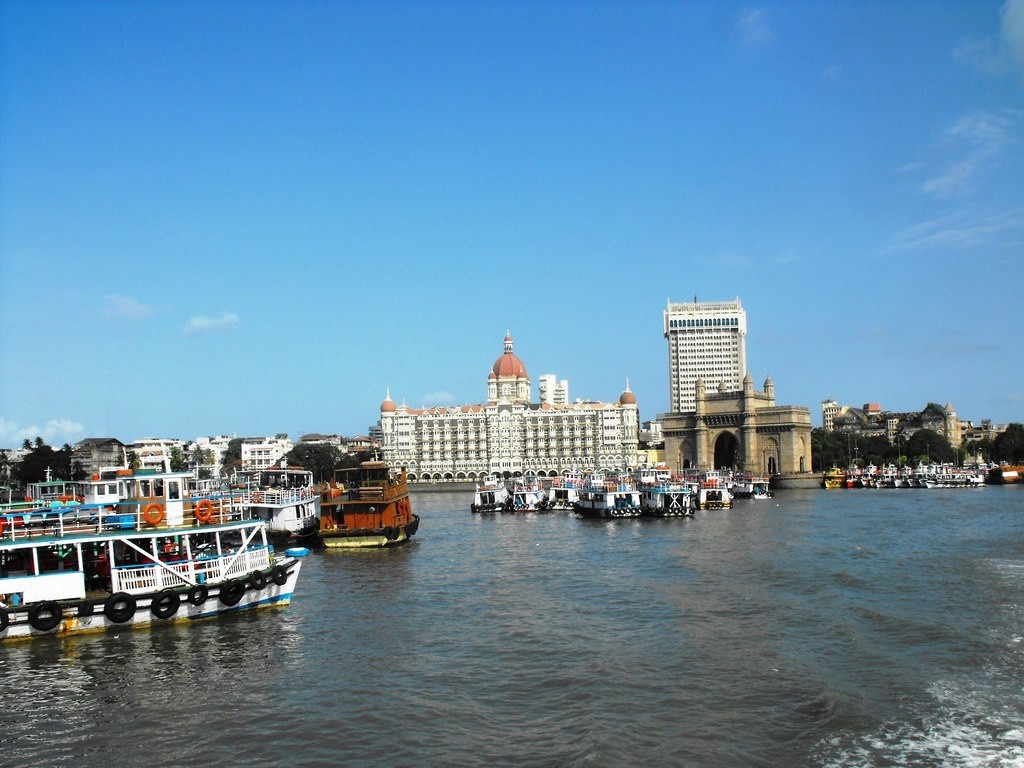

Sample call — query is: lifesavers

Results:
[526,487,531,492]
[517,487,522,492]
[144,502,163,524]
[398,502,405,512]
[196,500,212,522]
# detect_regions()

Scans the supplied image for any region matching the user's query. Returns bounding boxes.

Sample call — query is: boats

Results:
[823,455,1024,488]
[469,462,778,519]
[1,460,420,639]
